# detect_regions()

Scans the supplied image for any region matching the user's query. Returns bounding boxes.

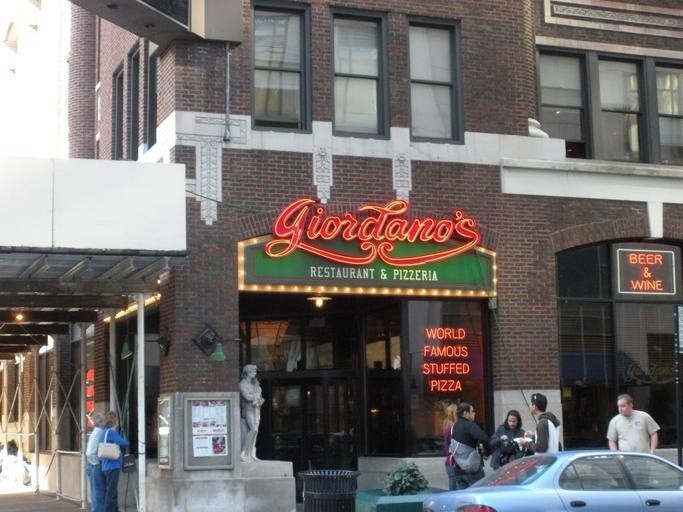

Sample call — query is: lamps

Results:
[118,324,173,359]
[192,323,227,362]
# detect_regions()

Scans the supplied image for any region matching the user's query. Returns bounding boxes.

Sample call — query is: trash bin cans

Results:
[298,470,361,512]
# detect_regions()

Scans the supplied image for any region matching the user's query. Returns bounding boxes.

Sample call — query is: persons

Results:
[604,393,662,457]
[239,364,265,465]
[101,410,130,512]
[442,403,464,490]
[489,409,535,471]
[85,414,109,512]
[518,392,561,455]
[450,402,489,489]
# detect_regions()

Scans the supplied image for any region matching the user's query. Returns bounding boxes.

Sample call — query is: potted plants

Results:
[356,458,450,512]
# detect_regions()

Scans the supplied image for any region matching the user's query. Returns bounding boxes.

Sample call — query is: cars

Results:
[376,451,683,512]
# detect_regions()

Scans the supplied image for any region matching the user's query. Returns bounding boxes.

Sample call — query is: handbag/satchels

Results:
[96,441,120,461]
[448,438,482,474]
[500,438,520,464]
[122,453,137,474]
